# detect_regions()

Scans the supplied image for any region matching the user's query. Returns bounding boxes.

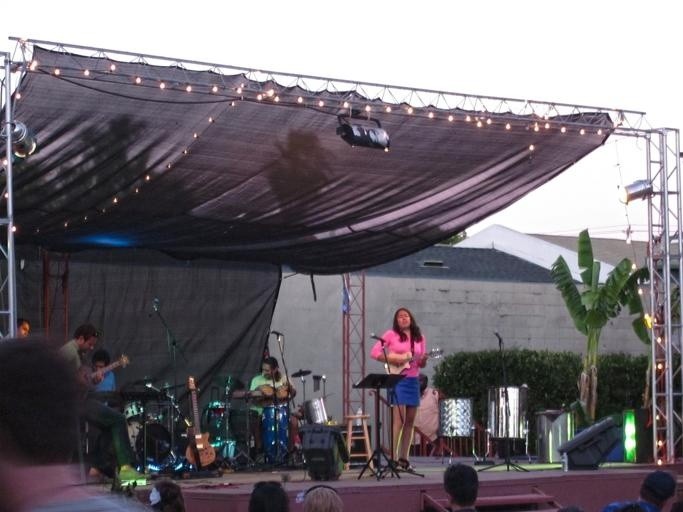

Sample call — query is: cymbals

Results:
[291,370,312,377]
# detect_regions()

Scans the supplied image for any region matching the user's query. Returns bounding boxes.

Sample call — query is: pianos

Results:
[90,391,170,405]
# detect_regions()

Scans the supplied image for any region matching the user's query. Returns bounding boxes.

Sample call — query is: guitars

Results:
[385,349,443,376]
[73,354,130,389]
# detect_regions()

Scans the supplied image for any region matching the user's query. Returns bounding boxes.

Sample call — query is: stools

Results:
[342,412,373,472]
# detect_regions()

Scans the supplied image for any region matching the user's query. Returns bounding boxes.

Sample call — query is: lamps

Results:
[335,107,391,153]
[618,179,653,204]
[621,407,651,464]
[0,119,37,160]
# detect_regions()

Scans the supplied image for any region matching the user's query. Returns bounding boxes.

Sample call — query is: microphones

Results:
[269,329,283,336]
[151,297,160,312]
[494,331,503,341]
[369,332,386,343]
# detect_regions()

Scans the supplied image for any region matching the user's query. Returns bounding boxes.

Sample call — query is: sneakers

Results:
[118,468,152,481]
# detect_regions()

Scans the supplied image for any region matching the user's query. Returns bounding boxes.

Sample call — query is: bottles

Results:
[355,408,363,426]
[561,451,568,472]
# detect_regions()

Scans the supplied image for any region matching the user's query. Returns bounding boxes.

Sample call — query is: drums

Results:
[254,384,275,408]
[208,402,232,429]
[184,377,216,467]
[275,385,290,405]
[129,423,170,465]
[302,396,331,425]
[230,407,258,464]
[123,399,146,422]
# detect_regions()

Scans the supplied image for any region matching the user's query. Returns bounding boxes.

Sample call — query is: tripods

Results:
[357,383,400,481]
[478,340,529,473]
[144,370,307,472]
[371,340,425,480]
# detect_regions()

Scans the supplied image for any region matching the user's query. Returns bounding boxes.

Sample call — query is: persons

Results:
[301,484,344,512]
[371,306,427,472]
[558,470,683,512]
[413,372,444,454]
[442,463,482,512]
[1,317,186,511]
[250,357,295,460]
[247,481,290,511]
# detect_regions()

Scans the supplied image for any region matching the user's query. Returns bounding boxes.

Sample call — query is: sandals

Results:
[394,459,416,472]
